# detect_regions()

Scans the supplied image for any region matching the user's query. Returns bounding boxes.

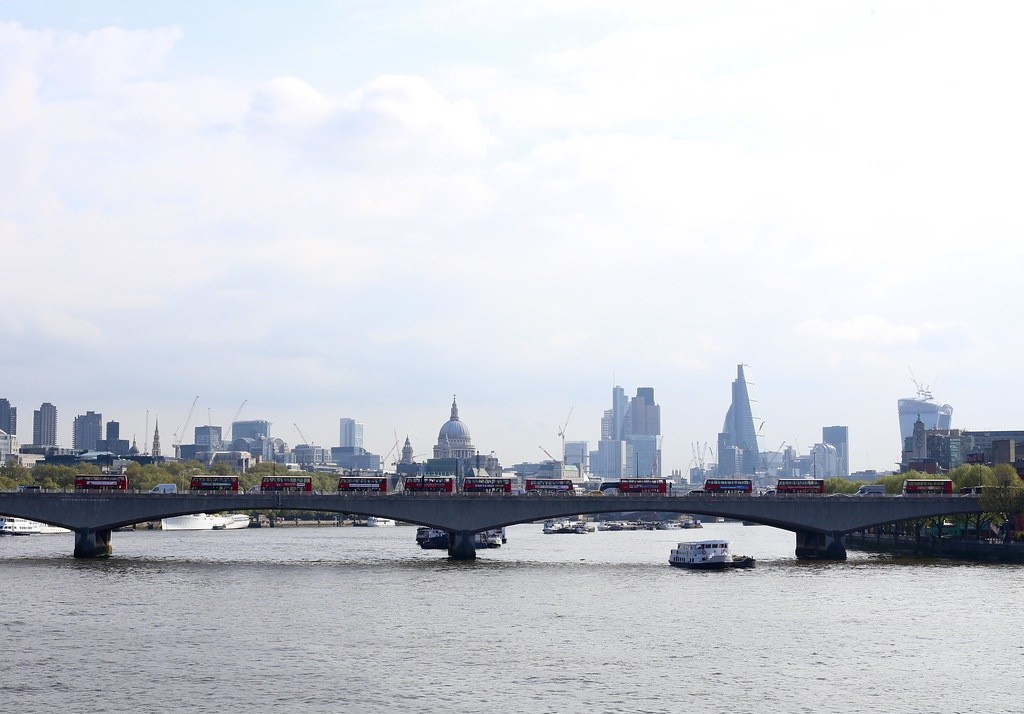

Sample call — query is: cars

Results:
[961,494,971,498]
[573,485,579,490]
[574,488,586,496]
[583,490,604,497]
[18,485,42,494]
[511,488,525,495]
[828,493,853,498]
[894,494,902,498]
[762,490,776,496]
[859,490,883,498]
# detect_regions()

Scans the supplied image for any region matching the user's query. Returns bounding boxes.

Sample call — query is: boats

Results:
[542,514,703,535]
[416,527,429,544]
[731,554,756,569]
[367,516,396,528]
[668,539,733,568]
[421,527,488,549]
[161,513,251,531]
[0,516,71,534]
[486,530,502,549]
[494,526,507,543]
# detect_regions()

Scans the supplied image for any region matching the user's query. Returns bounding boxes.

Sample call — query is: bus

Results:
[619,477,666,494]
[337,476,388,496]
[775,478,824,493]
[971,485,1016,499]
[260,475,313,496]
[462,477,512,497]
[704,479,752,494]
[524,478,576,497]
[404,475,455,496]
[75,474,129,495]
[598,481,620,496]
[188,475,240,496]
[902,478,953,498]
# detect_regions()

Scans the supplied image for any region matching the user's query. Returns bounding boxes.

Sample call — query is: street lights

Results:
[812,452,816,479]
[106,449,109,473]
[454,457,460,493]
[273,448,276,476]
[635,451,639,478]
[737,450,741,478]
[975,451,992,486]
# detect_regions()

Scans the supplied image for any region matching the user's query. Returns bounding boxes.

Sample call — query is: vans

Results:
[149,484,177,497]
[684,490,704,496]
[245,485,260,495]
[854,485,886,497]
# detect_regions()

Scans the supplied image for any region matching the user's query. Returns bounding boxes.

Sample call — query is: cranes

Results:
[557,406,574,462]
[380,427,426,472]
[173,395,200,458]
